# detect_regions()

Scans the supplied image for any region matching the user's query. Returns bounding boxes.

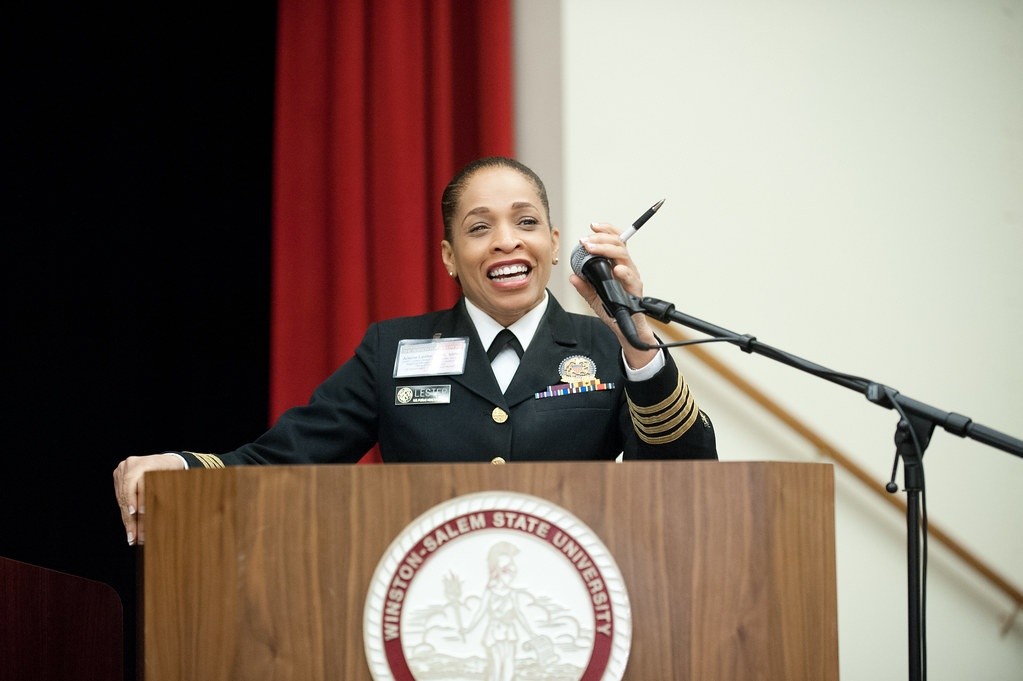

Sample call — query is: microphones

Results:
[570,242,640,347]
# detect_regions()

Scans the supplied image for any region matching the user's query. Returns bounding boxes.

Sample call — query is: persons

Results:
[113,156,719,547]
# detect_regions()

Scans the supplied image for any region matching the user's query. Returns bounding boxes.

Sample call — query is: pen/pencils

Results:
[619,198,667,244]
